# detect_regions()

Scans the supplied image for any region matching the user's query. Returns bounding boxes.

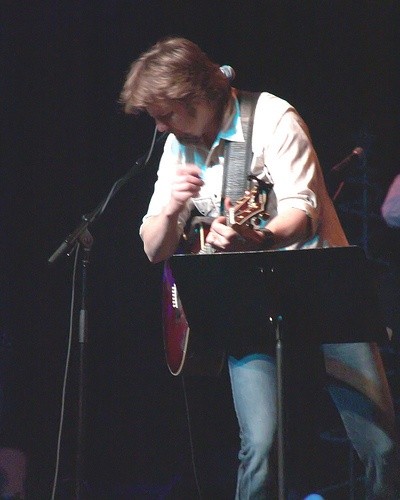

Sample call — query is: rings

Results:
[235,236,247,243]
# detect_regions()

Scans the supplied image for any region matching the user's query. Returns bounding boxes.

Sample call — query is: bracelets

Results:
[257,228,276,251]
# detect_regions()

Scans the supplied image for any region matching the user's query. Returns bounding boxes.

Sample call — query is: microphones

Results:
[219,65,236,79]
[329,146,363,173]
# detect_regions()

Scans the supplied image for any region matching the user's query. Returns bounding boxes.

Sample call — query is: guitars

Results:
[162,185,271,377]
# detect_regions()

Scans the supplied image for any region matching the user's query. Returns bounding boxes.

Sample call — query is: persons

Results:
[121,38,400,500]
